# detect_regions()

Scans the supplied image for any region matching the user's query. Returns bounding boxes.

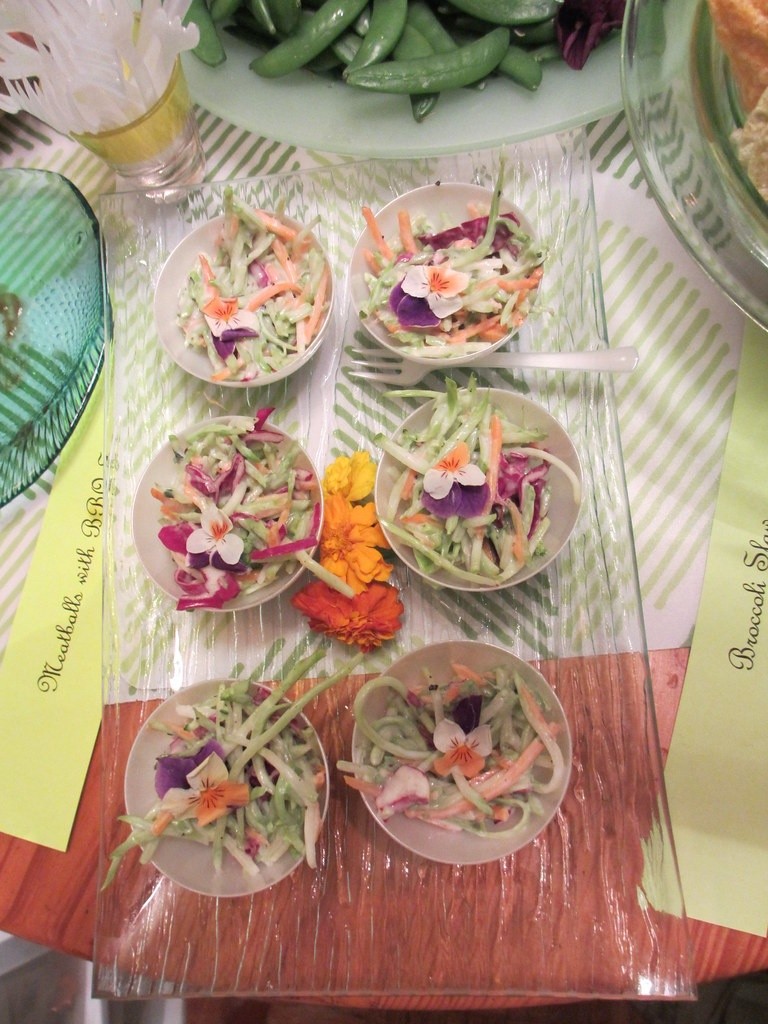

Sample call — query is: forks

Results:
[347,346,639,388]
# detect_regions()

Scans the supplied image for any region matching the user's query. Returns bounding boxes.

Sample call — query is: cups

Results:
[68,51,208,206]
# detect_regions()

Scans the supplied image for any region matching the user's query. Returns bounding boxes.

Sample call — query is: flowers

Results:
[159,752,251,826]
[289,447,406,655]
[186,510,245,565]
[423,442,487,500]
[432,718,492,778]
[401,265,471,319]
[200,296,261,338]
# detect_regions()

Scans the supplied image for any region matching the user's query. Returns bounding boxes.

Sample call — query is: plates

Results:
[179,0,671,158]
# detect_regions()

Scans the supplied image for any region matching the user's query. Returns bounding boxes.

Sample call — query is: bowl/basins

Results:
[351,640,574,866]
[0,166,117,512]
[132,415,324,612]
[375,389,584,592]
[620,0,768,332]
[347,183,540,367]
[124,678,329,897]
[153,209,334,387]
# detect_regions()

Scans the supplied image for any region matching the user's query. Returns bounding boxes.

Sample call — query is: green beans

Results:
[182,0,561,122]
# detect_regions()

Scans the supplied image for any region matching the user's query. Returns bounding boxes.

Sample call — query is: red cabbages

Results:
[556,0,627,69]
